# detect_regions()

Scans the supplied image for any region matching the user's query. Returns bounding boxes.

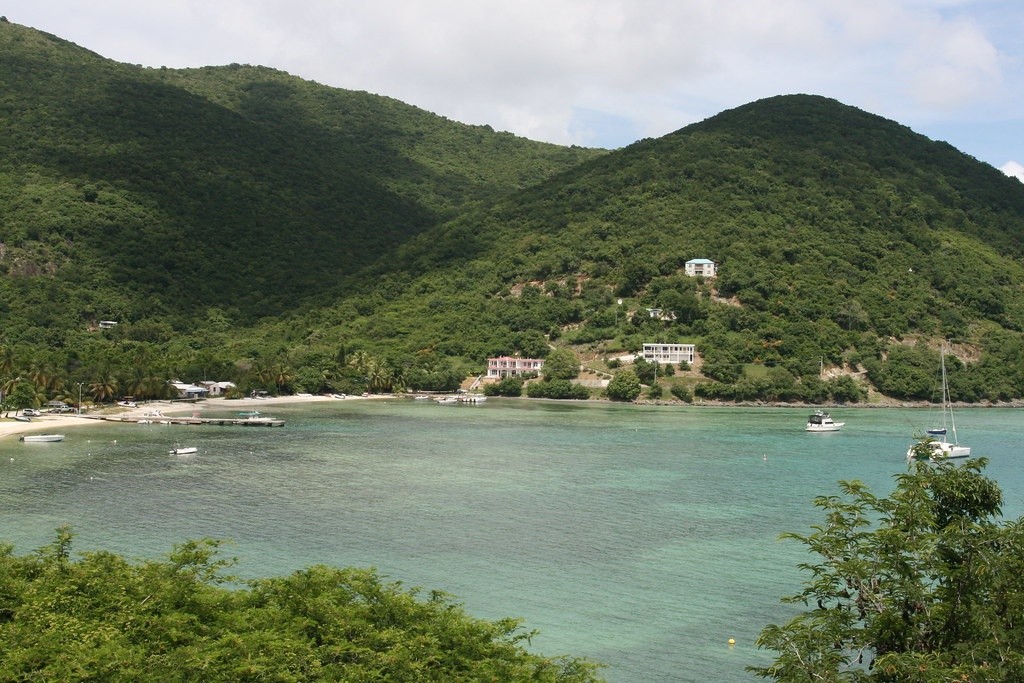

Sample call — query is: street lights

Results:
[77,382,85,414]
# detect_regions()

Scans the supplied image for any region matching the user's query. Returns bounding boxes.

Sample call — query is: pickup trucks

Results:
[54,405,75,414]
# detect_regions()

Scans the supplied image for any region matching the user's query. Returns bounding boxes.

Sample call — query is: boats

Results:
[414,391,488,404]
[805,404,846,433]
[169,447,198,454]
[925,426,948,435]
[24,433,66,442]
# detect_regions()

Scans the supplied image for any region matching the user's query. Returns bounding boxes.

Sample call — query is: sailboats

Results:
[906,345,972,460]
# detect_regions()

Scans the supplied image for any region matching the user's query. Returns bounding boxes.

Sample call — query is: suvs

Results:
[23,408,41,418]
